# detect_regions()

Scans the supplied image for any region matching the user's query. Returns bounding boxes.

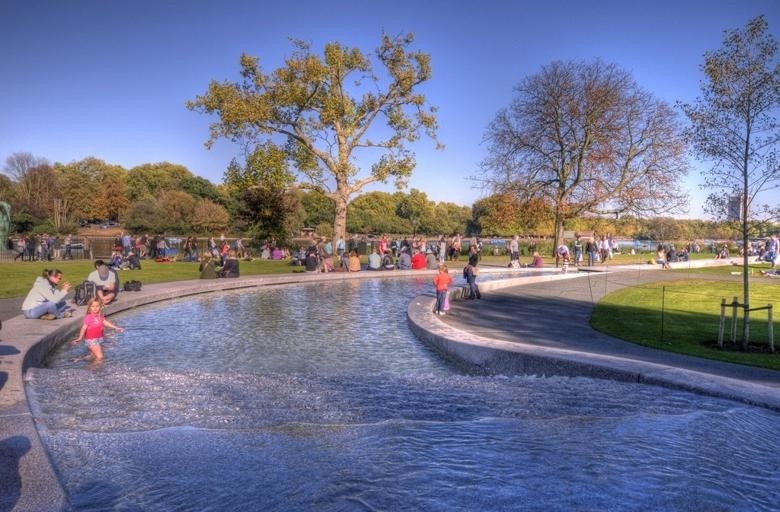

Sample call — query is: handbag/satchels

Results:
[75,281,97,306]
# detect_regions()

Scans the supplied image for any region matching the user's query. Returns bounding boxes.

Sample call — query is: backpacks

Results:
[124,280,141,291]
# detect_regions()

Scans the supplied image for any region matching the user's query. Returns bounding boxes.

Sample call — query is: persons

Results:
[93,259,121,300]
[86,264,116,303]
[71,297,124,360]
[21,269,72,320]
[432,263,453,316]
[7,232,779,278]
[463,245,482,300]
[83,361,109,372]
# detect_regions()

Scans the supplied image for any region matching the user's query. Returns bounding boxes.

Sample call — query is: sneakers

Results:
[40,314,56,320]
[64,312,72,318]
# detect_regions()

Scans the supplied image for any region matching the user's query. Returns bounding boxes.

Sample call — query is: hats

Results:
[98,265,109,281]
